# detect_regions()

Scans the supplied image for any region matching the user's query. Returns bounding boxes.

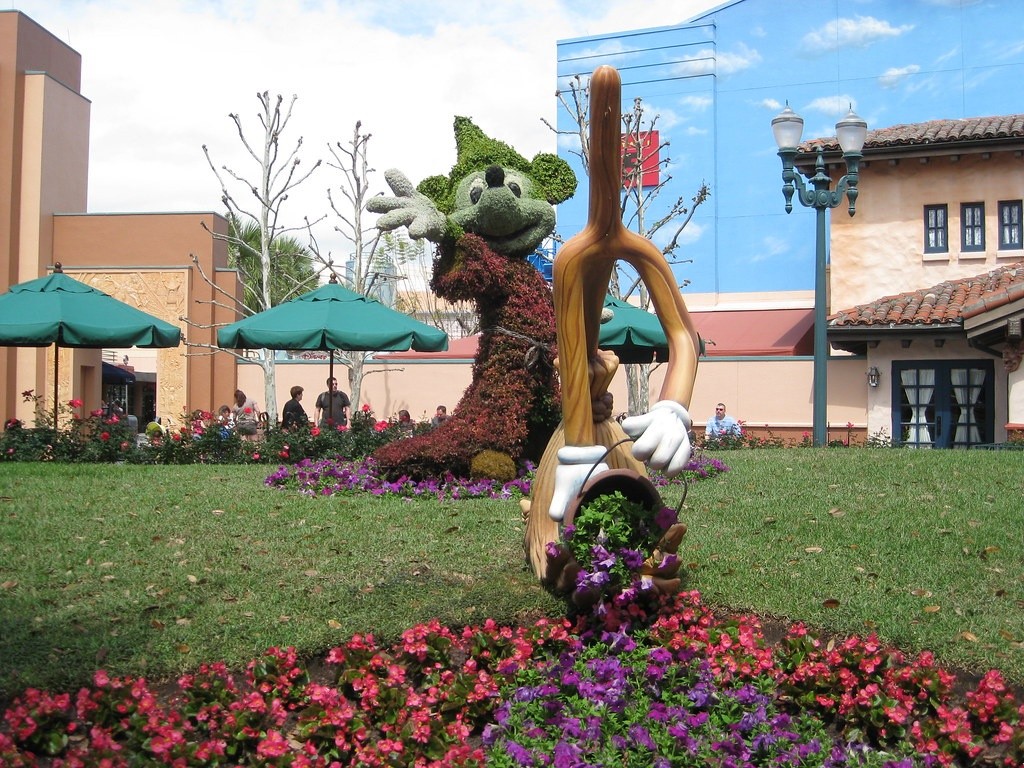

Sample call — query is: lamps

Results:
[868,368,881,387]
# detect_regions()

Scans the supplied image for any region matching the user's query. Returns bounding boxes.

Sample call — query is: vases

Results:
[557,437,688,535]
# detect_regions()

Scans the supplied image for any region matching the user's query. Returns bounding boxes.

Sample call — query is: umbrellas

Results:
[0,273,181,429]
[216,280,449,432]
[101,361,135,385]
[595,296,707,363]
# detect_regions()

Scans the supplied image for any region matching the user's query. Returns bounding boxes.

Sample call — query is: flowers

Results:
[546,491,678,615]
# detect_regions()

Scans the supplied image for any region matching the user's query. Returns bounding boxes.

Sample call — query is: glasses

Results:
[333,383,337,385]
[716,408,723,411]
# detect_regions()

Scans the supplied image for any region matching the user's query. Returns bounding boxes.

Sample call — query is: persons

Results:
[281,386,308,429]
[706,403,741,443]
[399,410,413,424]
[190,390,262,441]
[113,399,123,417]
[314,377,352,429]
[430,406,451,428]
